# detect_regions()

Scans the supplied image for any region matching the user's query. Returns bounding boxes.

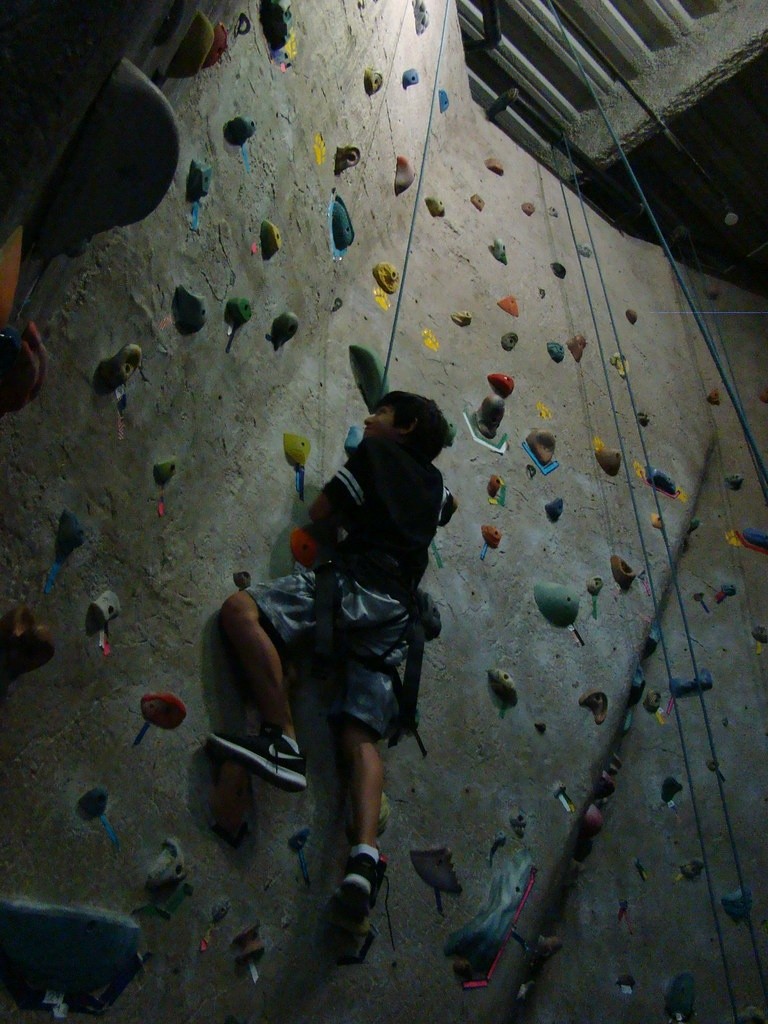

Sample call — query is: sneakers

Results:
[334,844,377,917]
[211,722,307,792]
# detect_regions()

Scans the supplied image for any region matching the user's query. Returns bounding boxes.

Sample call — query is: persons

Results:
[209,391,458,911]
